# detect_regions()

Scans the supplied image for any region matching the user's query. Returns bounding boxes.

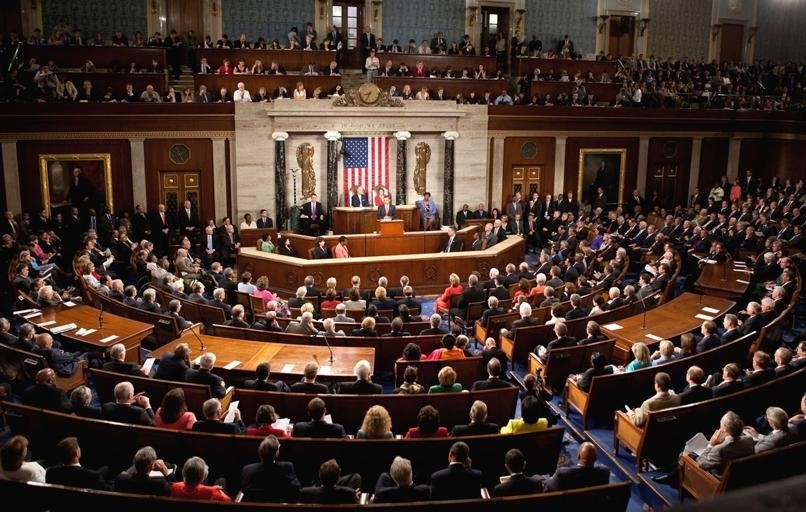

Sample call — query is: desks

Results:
[0,40,806,133]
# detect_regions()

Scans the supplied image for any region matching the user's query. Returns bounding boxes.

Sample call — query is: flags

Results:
[341,136,390,205]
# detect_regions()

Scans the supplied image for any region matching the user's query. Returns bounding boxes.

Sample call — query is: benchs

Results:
[1,197,805,512]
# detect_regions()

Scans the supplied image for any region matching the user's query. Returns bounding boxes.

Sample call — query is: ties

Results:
[312,204,315,221]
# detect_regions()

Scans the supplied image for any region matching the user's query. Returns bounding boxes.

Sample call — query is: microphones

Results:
[641,305,648,330]
[189,327,204,351]
[320,331,334,361]
[98,304,104,328]
